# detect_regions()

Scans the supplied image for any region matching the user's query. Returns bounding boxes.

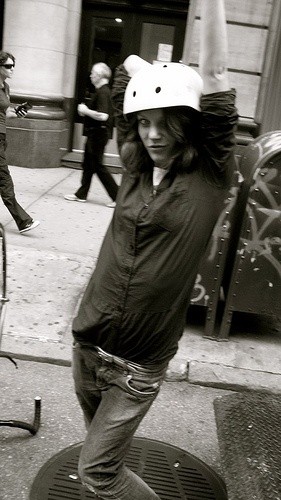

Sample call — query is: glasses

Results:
[0,64,14,69]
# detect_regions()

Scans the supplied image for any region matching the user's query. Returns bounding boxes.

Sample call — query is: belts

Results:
[94,346,160,373]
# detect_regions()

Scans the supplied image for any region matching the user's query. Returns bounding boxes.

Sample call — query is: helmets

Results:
[123,44,203,114]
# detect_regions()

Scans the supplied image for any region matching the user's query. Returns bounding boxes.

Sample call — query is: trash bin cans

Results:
[191,129,281,342]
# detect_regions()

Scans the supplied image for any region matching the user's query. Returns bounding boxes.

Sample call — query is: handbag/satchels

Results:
[82,115,108,139]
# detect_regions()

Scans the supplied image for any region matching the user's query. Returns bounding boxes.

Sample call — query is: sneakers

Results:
[19,222,40,233]
[107,202,117,208]
[65,194,87,202]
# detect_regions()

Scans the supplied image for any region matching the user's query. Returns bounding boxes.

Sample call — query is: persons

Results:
[63,62,120,207]
[72,0,236,499]
[0,51,42,232]
